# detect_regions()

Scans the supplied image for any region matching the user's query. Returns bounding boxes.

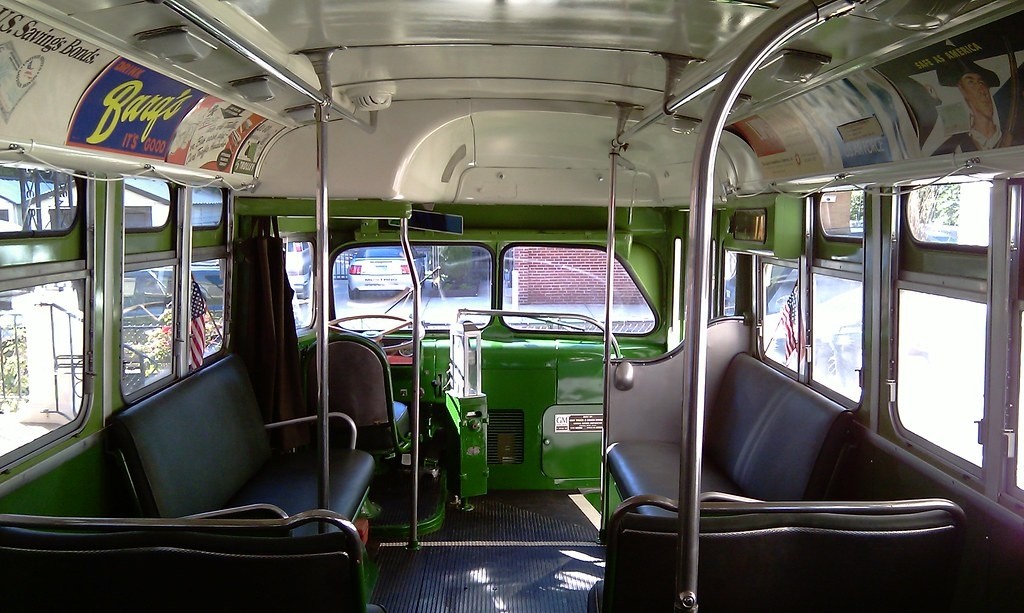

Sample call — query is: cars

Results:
[765,271,848,379]
[346,246,427,298]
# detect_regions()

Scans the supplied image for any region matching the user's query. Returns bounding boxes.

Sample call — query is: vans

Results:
[126,237,313,342]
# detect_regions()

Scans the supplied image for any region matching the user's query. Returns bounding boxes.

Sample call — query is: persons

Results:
[936,57,1021,156]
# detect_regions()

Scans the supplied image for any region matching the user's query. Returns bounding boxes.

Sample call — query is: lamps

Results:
[132,24,316,125]
[662,0,976,134]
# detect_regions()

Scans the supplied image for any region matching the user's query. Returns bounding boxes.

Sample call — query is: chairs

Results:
[302,333,424,472]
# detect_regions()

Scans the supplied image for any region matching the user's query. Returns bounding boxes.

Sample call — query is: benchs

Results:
[605,350,854,518]
[113,353,376,536]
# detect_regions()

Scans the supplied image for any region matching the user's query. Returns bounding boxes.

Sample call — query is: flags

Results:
[781,277,806,368]
[188,280,207,371]
